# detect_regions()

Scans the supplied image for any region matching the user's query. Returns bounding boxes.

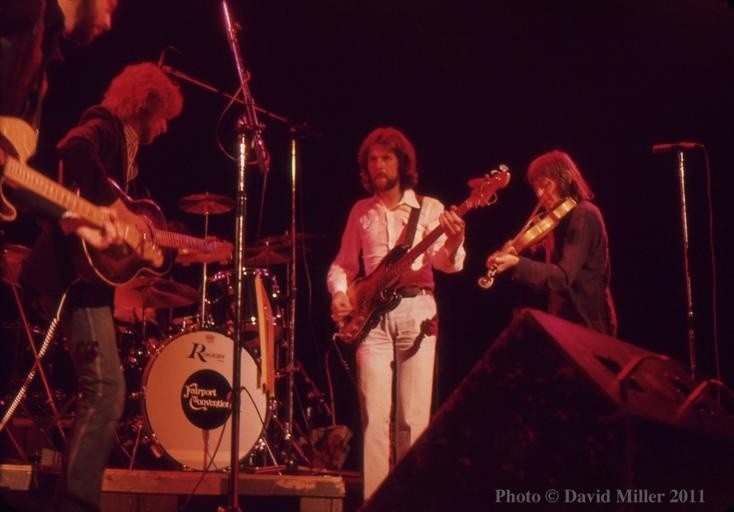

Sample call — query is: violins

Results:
[477,196,577,290]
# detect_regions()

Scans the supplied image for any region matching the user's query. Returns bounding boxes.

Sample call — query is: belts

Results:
[393,284,435,298]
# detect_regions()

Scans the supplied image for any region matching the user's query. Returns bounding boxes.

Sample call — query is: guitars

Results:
[331,164,511,346]
[0,117,165,268]
[71,177,234,287]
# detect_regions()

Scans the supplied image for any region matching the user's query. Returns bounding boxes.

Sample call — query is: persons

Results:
[323,124,469,506]
[484,148,619,339]
[46,61,187,511]
[0,1,123,413]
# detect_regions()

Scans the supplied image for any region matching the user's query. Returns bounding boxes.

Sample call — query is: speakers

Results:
[357,308,734,511]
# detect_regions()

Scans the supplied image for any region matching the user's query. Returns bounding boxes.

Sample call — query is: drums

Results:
[200,267,289,352]
[118,321,268,472]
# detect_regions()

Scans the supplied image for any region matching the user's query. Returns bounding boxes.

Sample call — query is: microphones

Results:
[158,48,168,68]
[650,140,704,160]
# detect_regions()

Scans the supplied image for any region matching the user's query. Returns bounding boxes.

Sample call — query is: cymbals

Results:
[244,232,326,267]
[176,192,235,215]
